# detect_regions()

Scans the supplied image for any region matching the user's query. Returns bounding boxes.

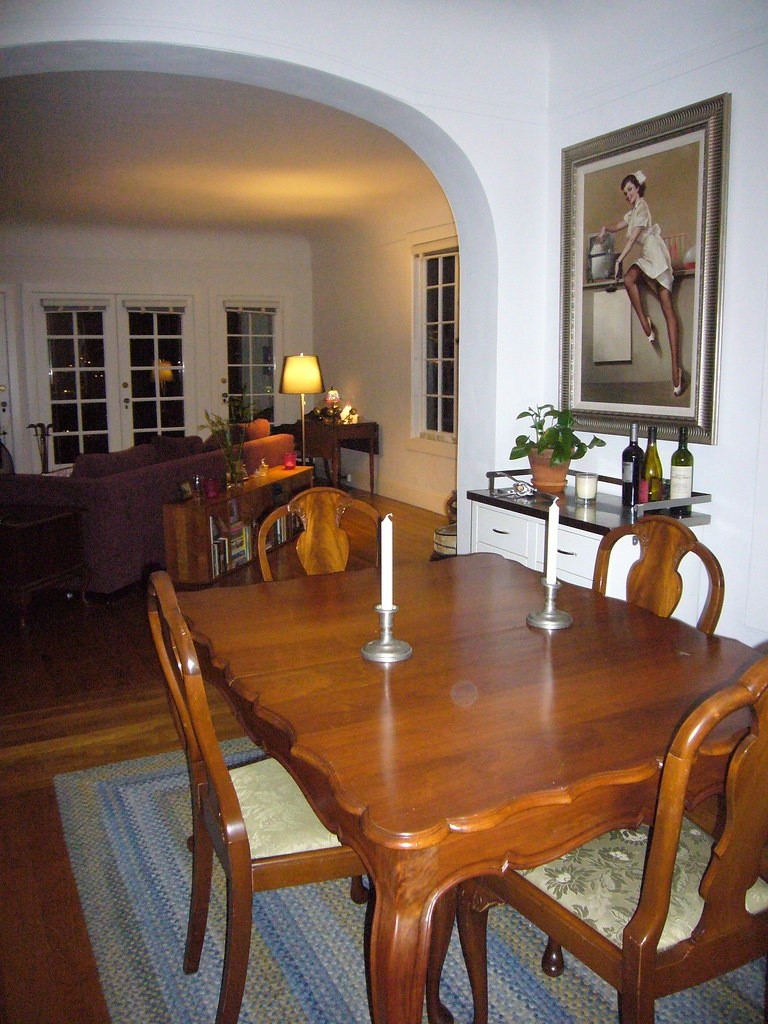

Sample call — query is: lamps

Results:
[277,352,325,467]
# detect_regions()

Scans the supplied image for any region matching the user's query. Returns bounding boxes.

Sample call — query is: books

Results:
[228,525,253,569]
[210,516,229,578]
[265,515,292,550]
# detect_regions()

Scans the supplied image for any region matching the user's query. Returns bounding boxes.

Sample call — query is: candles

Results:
[546,498,560,585]
[381,512,394,609]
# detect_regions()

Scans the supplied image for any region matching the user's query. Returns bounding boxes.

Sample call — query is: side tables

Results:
[0,502,92,634]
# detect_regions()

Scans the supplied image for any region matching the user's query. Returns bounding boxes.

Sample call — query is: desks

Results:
[175,552,768,1024]
[304,421,379,496]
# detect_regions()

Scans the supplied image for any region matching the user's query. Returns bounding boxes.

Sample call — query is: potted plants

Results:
[511,402,605,493]
[196,384,258,492]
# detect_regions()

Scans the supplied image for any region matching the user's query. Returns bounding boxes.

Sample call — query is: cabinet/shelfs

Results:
[469,502,641,602]
[162,463,315,586]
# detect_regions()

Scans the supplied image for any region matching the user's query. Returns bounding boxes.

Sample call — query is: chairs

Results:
[256,485,383,583]
[147,570,369,1024]
[456,657,768,1024]
[591,516,729,640]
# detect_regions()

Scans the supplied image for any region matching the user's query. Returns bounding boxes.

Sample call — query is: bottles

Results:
[622,421,644,506]
[669,427,693,518]
[193,475,203,505]
[638,427,663,515]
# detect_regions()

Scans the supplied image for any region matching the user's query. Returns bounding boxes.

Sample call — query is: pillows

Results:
[71,417,272,479]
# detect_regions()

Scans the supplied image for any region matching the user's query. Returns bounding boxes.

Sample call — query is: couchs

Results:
[1,421,296,602]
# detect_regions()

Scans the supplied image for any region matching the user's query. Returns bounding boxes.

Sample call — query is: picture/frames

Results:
[558,92,731,447]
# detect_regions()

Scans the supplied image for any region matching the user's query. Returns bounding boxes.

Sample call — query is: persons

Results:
[595,169,682,398]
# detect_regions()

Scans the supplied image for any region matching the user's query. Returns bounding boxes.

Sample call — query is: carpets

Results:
[53,734,768,1024]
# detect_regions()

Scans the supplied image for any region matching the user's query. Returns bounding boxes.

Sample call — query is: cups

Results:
[258,461,269,476]
[574,472,598,505]
[284,452,297,469]
[204,478,221,498]
